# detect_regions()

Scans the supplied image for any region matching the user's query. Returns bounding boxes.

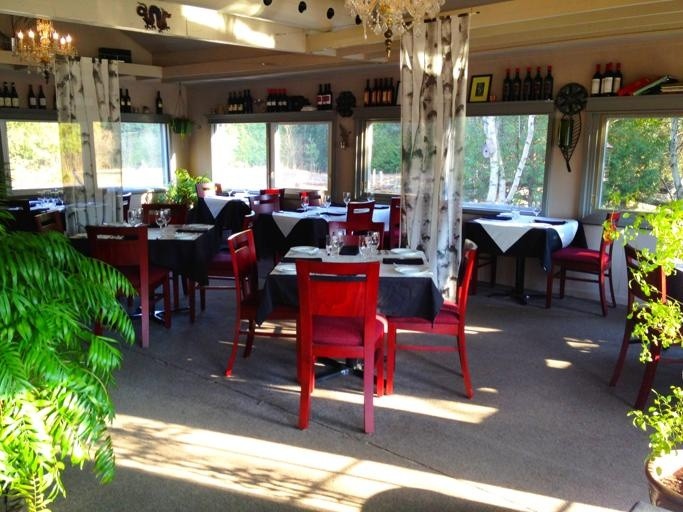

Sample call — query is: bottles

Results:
[125,88,131,112]
[362,77,394,106]
[119,88,125,113]
[27,84,36,109]
[155,90,163,114]
[53,86,56,109]
[601,62,621,95]
[0,80,11,107]
[501,65,553,100]
[227,88,252,113]
[590,61,601,95]
[265,88,288,112]
[10,82,20,108]
[316,83,332,110]
[38,85,46,109]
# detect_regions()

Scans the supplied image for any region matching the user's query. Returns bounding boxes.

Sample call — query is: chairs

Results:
[260,188,285,210]
[83,223,172,348]
[389,196,400,247]
[140,204,190,310]
[36,209,66,236]
[196,182,223,198]
[113,192,132,223]
[294,258,385,435]
[2,199,33,231]
[608,242,683,411]
[325,220,386,253]
[463,249,497,295]
[346,200,378,236]
[248,194,283,214]
[226,228,298,377]
[543,211,620,316]
[299,190,326,206]
[388,239,479,399]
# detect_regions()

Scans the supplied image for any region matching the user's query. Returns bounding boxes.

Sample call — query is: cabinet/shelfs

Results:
[0,106,171,124]
[203,110,336,126]
[583,93,683,112]
[351,99,554,121]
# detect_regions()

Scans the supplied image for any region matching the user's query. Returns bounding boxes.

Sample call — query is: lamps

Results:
[347,1,450,60]
[8,18,79,78]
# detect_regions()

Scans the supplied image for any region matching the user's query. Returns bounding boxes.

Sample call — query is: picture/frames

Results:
[467,74,493,103]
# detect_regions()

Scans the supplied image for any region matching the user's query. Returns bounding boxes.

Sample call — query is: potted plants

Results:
[601,172,683,510]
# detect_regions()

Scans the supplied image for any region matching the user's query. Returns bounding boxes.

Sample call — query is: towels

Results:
[482,216,512,220]
[339,245,359,255]
[147,223,168,228]
[330,203,346,207]
[374,207,389,209]
[535,220,566,225]
[321,212,346,216]
[292,210,304,212]
[280,257,322,263]
[176,228,208,233]
[383,257,424,265]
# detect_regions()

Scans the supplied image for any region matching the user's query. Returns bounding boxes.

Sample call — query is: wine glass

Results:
[41,191,64,209]
[358,236,372,263]
[367,190,375,202]
[323,195,331,212]
[155,211,165,233]
[330,229,344,261]
[532,204,541,220]
[301,197,309,216]
[160,209,171,232]
[128,208,144,227]
[368,232,380,257]
[343,192,351,211]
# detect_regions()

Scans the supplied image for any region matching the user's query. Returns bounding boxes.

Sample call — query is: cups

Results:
[511,208,520,222]
[325,235,330,256]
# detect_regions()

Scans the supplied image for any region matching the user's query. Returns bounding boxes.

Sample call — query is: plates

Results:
[390,248,418,256]
[274,265,295,272]
[290,246,318,255]
[394,266,428,274]
